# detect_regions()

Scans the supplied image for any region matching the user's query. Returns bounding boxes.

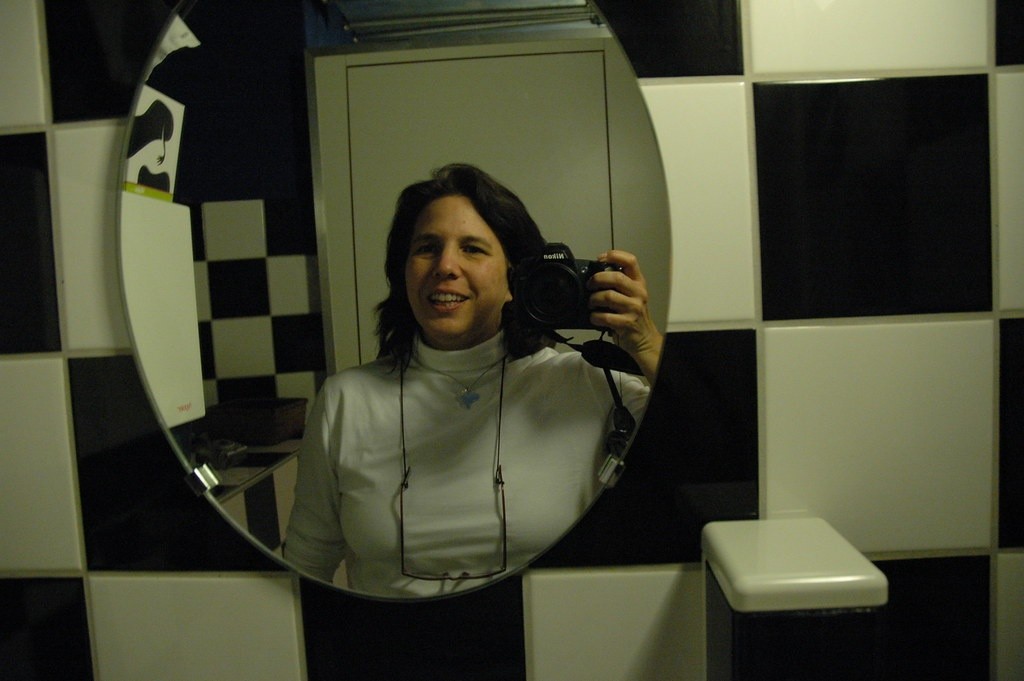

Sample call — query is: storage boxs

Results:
[208,398,307,444]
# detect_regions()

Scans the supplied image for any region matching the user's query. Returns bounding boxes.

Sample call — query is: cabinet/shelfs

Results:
[120,191,206,430]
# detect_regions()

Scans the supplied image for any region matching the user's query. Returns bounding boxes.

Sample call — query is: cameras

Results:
[504,242,622,331]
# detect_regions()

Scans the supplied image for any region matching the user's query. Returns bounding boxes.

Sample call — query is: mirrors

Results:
[112,3,677,606]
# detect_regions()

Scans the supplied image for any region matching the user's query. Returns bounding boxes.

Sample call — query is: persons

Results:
[283,163,664,597]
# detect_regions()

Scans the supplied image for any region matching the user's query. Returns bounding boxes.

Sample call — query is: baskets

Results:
[206,397,308,447]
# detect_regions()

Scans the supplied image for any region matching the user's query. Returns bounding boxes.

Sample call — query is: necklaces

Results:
[413,358,501,409]
